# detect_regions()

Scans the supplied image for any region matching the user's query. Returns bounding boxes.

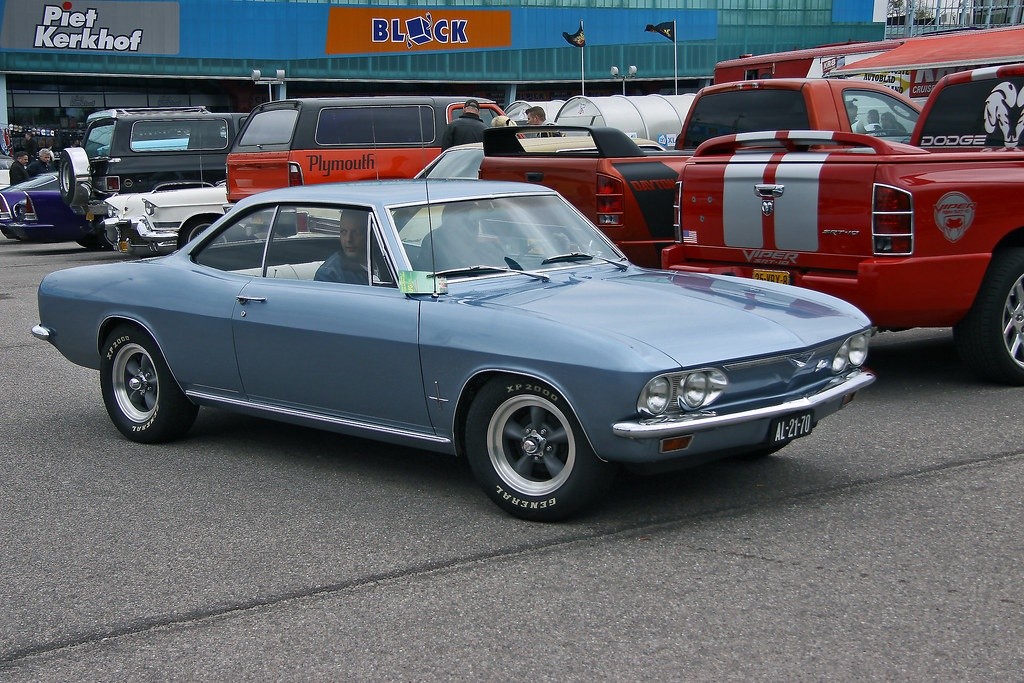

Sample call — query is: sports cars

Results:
[26,175,881,527]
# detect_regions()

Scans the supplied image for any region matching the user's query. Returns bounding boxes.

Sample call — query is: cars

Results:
[100,179,242,254]
[0,156,114,252]
[390,133,668,247]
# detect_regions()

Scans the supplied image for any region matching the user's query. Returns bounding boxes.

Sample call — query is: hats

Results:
[464,99,479,112]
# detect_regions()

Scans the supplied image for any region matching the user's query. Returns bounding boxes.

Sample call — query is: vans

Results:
[222,95,528,238]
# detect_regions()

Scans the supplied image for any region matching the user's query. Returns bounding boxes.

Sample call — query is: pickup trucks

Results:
[657,62,1024,390]
[478,77,924,269]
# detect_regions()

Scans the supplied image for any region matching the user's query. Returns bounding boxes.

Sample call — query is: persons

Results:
[417,203,506,271]
[441,99,562,154]
[845,103,907,137]
[9,132,81,186]
[314,208,392,286]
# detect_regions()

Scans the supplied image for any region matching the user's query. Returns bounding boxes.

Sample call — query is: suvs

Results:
[58,105,253,225]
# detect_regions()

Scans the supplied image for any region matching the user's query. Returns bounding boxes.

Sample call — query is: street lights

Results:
[251,68,286,102]
[610,65,638,96]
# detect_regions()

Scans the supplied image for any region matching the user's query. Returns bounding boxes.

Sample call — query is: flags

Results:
[562,19,586,47]
[644,20,677,43]
